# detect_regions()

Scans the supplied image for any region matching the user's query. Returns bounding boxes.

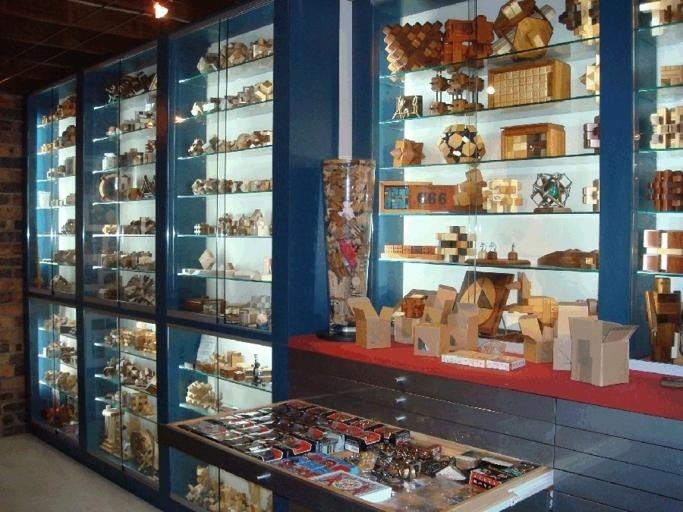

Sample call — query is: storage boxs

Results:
[344,287,636,387]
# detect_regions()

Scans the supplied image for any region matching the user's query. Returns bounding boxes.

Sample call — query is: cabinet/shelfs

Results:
[364,1,602,342]
[633,0,682,380]
[83,44,157,510]
[23,74,83,447]
[169,1,277,512]
[163,398,552,511]
